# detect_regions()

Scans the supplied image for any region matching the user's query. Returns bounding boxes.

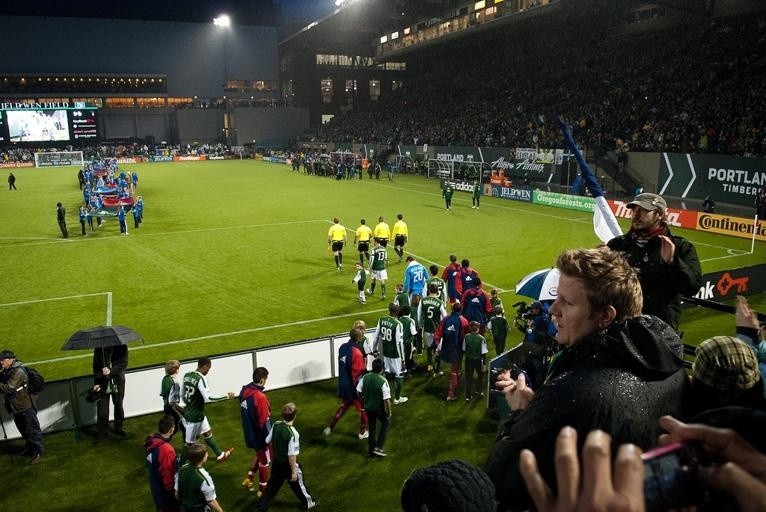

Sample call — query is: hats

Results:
[0,350,14,360]
[691,333,761,390]
[626,193,666,214]
[531,302,541,308]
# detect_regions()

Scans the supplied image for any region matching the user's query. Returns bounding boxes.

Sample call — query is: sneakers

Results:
[216,447,234,463]
[372,446,387,457]
[445,392,484,403]
[432,372,443,379]
[302,497,320,511]
[393,397,408,405]
[322,427,331,436]
[17,447,30,456]
[241,479,256,492]
[427,365,434,374]
[357,431,368,440]
[30,452,40,464]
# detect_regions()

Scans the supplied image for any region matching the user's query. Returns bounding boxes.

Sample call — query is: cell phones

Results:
[642,439,728,512]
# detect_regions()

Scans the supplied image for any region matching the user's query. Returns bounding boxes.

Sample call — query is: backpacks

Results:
[23,367,44,395]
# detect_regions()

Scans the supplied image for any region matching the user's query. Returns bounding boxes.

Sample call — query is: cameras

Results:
[85,389,106,403]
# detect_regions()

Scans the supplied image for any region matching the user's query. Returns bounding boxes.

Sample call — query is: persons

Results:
[4,141,307,161]
[144,357,322,512]
[93,343,128,444]
[289,7,765,210]
[57,154,144,236]
[401,193,765,511]
[8,173,17,190]
[1,350,44,464]
[324,213,510,456]
